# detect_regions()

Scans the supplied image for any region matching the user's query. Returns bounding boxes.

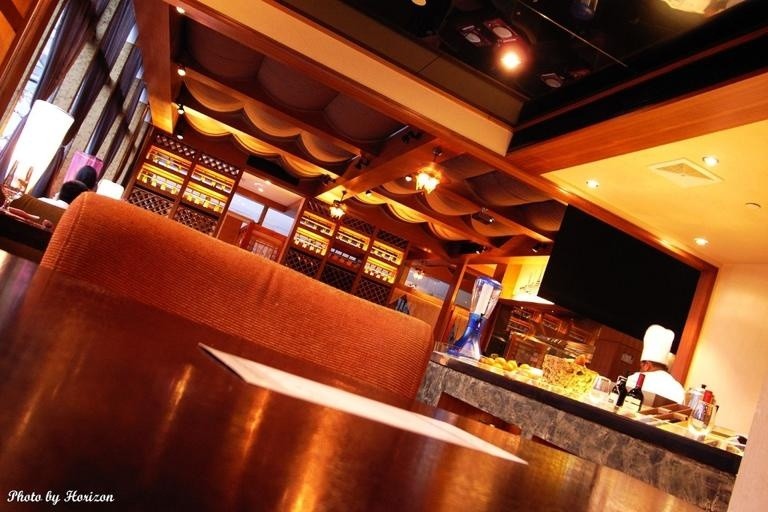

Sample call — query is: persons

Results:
[37,165,96,210]
[625,361,685,406]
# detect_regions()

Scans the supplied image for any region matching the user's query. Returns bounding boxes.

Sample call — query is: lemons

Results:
[479,353,531,377]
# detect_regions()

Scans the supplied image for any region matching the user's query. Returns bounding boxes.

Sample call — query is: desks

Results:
[0,248,705,512]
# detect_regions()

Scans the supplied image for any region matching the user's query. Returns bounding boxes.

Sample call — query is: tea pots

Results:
[689,384,709,410]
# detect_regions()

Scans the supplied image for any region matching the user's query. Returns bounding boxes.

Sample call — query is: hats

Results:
[640,324,676,366]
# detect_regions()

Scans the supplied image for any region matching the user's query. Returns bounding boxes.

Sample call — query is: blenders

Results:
[451,273,502,360]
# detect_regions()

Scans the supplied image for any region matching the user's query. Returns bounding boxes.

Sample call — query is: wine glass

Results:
[688,399,715,433]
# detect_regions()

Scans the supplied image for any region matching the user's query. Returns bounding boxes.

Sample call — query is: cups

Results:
[593,374,611,392]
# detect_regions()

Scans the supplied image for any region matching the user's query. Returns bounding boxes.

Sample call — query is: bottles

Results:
[609,374,646,413]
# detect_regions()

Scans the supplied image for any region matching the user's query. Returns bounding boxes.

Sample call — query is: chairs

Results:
[0,179,436,400]
